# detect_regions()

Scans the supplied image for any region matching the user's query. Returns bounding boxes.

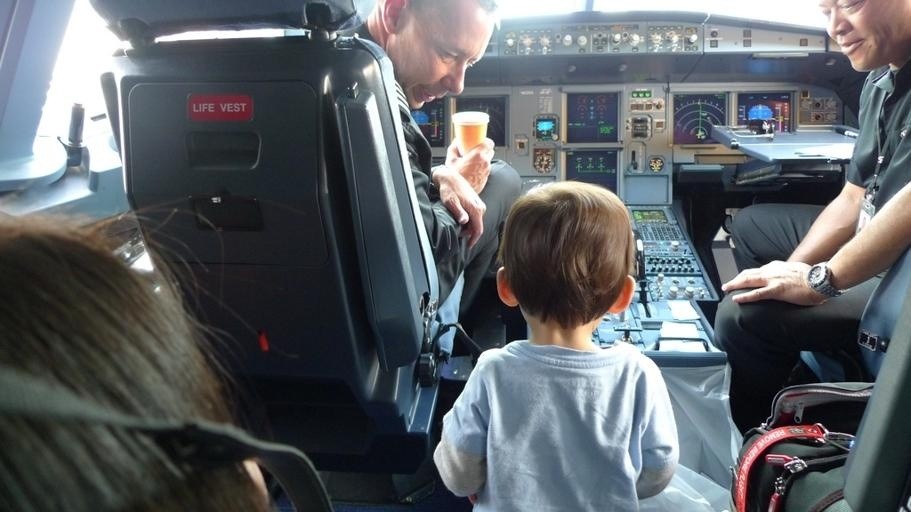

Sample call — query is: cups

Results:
[452,111,490,158]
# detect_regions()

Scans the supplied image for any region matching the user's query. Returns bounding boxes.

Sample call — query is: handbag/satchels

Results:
[729,382,875,511]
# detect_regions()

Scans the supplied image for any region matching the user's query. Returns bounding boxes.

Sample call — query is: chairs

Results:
[730,246,911,511]
[91,0,464,476]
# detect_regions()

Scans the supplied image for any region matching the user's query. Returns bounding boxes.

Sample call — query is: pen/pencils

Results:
[836,127,859,139]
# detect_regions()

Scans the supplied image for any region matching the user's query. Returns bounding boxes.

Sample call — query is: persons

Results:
[342,0,524,310]
[713,0,911,423]
[433,180,679,512]
[0,219,276,512]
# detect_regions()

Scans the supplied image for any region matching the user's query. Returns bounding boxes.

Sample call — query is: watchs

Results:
[807,262,844,297]
